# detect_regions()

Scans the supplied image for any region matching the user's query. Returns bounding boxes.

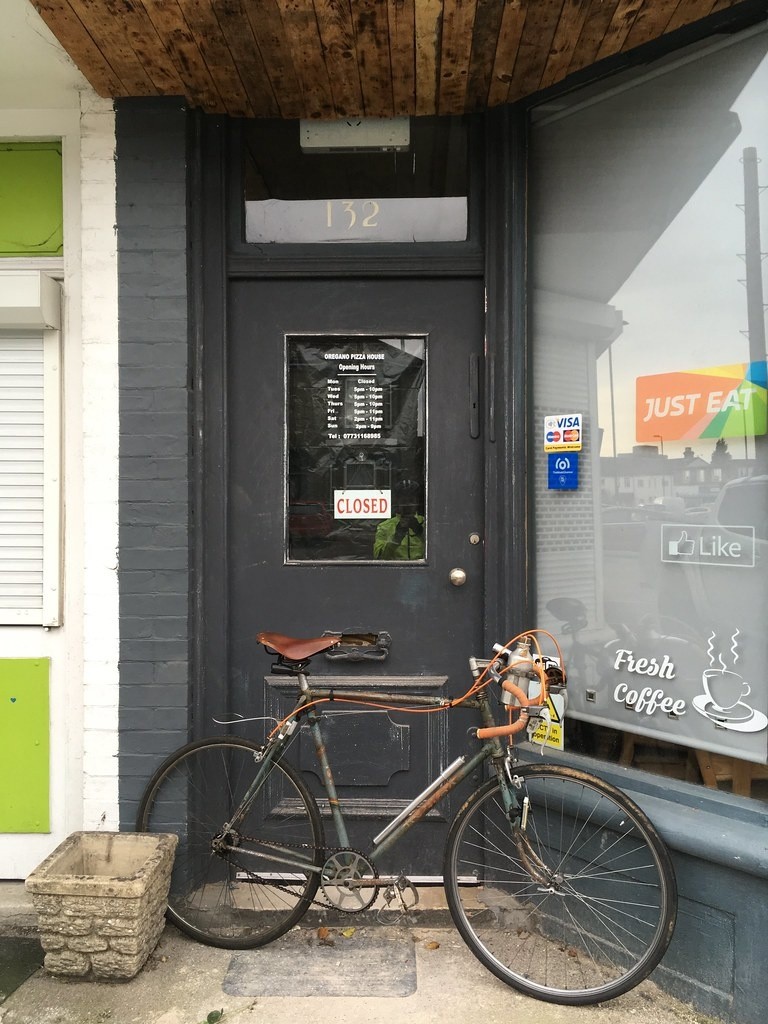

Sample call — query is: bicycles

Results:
[546,596,605,692]
[132,629,682,1008]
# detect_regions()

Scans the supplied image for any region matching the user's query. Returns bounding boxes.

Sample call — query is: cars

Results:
[700,474,768,579]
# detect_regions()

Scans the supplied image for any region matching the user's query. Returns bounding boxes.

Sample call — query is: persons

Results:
[370,477,427,562]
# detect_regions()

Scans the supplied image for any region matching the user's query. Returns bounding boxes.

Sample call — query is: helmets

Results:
[394,480,420,496]
